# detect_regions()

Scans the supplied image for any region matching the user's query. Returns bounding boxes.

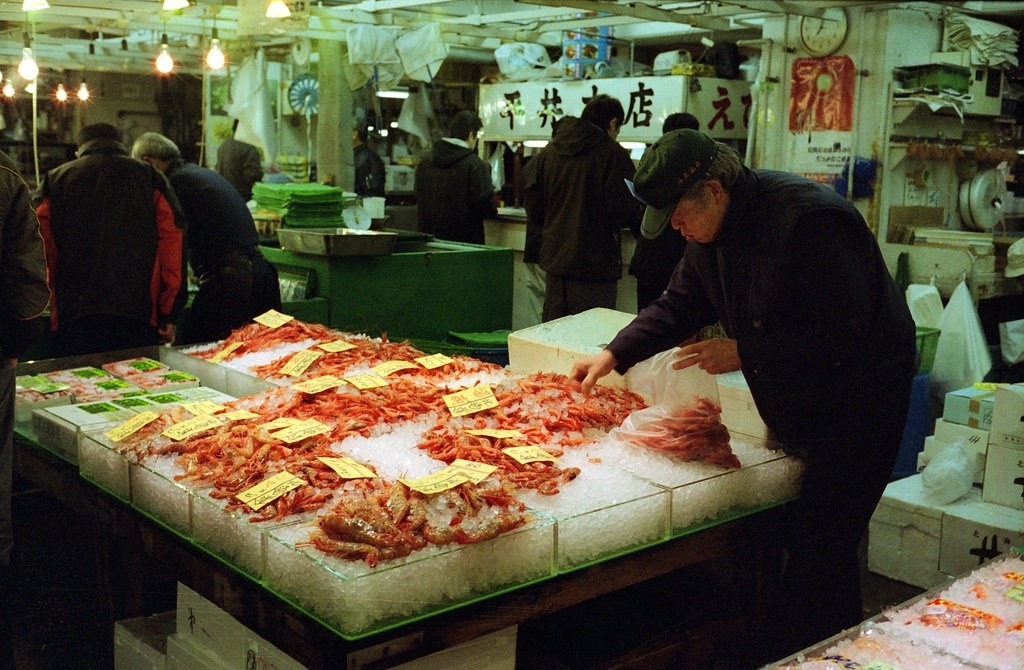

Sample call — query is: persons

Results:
[218,120,262,198]
[32,123,187,354]
[627,113,700,317]
[131,133,279,342]
[570,130,918,638]
[415,111,497,245]
[1,152,51,595]
[352,118,385,199]
[522,95,636,324]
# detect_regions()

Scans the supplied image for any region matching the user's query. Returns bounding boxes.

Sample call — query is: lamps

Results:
[163,0,190,11]
[266,1,291,17]
[22,0,50,10]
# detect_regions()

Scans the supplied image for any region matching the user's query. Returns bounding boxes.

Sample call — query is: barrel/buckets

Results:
[364,197,385,219]
[342,192,358,207]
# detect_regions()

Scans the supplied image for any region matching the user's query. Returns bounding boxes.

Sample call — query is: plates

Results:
[343,207,372,230]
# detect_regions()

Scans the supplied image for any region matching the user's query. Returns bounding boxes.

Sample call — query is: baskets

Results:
[916,327,942,374]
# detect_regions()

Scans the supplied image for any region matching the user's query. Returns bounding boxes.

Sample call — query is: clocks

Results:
[797,5,849,57]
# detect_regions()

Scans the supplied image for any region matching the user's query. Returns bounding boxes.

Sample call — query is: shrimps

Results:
[112,317,743,569]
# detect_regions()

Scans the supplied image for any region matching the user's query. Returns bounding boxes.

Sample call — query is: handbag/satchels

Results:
[616,344,743,470]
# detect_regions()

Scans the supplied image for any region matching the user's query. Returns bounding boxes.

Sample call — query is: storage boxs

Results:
[561,8,613,78]
[377,154,415,193]
[901,66,976,92]
[0,306,1024,670]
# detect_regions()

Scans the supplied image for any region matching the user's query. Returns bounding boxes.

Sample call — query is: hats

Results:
[77,122,122,147]
[633,129,719,240]
